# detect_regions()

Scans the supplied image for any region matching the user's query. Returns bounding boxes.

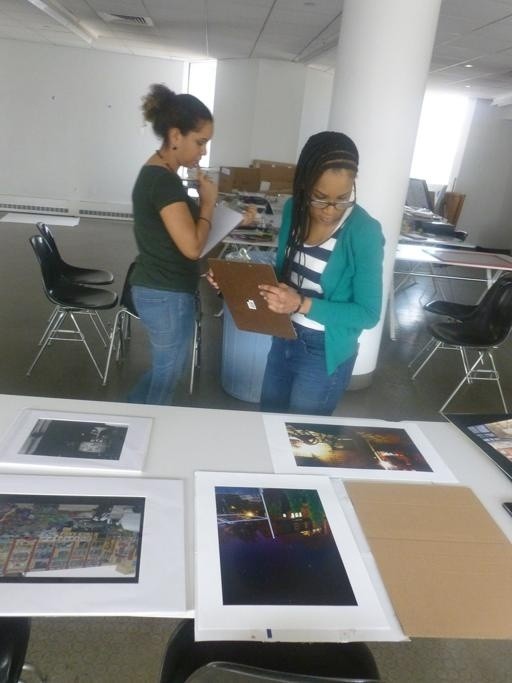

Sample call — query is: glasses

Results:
[306,179,356,210]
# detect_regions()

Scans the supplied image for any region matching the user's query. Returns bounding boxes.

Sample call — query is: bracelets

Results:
[294,291,305,312]
[198,216,212,228]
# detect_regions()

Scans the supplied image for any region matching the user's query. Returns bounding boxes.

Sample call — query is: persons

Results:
[126,84,219,406]
[205,130,385,416]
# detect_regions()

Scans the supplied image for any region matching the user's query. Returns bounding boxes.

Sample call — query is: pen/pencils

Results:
[196,163,219,208]
[199,272,210,279]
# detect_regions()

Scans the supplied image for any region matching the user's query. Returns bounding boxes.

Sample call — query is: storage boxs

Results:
[220,159,296,197]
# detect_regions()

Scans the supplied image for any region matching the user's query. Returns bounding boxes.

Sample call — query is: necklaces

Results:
[156,149,173,171]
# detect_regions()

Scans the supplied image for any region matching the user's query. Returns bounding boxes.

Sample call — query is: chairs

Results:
[26,221,202,396]
[405,271,511,415]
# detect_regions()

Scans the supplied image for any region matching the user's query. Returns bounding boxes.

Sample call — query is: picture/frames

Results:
[185,467,410,646]
[1,410,155,474]
[1,477,185,616]
[264,412,455,485]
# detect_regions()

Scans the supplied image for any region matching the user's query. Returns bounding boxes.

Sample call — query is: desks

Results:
[0,392,512,627]
[394,206,512,304]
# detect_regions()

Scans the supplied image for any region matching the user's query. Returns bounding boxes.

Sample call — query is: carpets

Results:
[1,212,80,226]
